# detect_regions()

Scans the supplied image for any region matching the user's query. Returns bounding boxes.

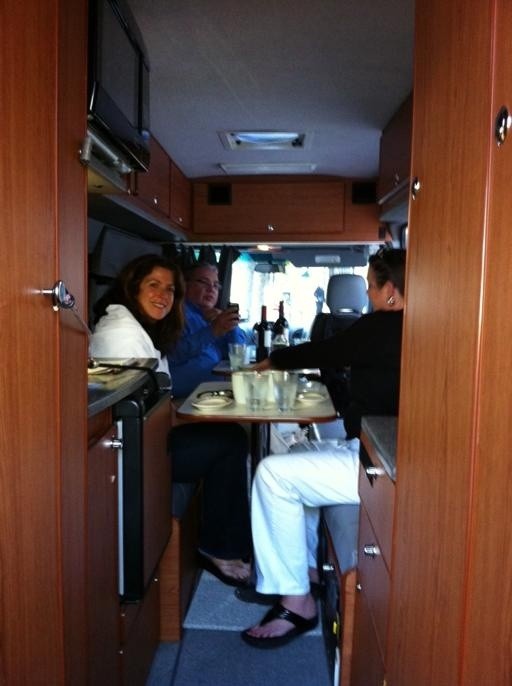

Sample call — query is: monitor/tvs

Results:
[88,0,150,173]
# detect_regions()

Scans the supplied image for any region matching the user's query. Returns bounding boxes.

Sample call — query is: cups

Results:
[228,303,239,320]
[229,343,250,369]
[232,370,299,414]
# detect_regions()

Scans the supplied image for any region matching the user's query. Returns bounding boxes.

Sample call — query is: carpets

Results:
[182,568,323,637]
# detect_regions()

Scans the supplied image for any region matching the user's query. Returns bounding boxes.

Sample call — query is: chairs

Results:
[308,273,367,340]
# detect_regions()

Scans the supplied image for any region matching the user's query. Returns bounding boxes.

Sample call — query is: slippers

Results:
[241,605,318,648]
[196,549,253,587]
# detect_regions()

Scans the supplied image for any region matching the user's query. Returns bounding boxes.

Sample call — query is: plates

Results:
[191,395,231,411]
[297,392,328,406]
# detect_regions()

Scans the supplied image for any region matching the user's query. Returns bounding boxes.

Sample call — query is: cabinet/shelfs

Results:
[374,90,412,220]
[79,378,173,686]
[0,1,89,685]
[133,131,191,235]
[187,176,391,244]
[345,434,394,685]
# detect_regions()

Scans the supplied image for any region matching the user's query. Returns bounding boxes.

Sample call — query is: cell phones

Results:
[228,302,240,322]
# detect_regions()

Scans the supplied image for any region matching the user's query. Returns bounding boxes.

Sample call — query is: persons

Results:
[239,245,408,648]
[87,253,254,584]
[164,262,250,396]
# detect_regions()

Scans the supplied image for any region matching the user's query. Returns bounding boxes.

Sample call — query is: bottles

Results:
[256,301,290,361]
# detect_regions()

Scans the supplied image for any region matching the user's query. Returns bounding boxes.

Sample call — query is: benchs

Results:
[312,499,359,686]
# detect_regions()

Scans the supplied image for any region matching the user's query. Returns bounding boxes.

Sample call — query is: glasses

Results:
[197,390,232,399]
[186,278,222,289]
